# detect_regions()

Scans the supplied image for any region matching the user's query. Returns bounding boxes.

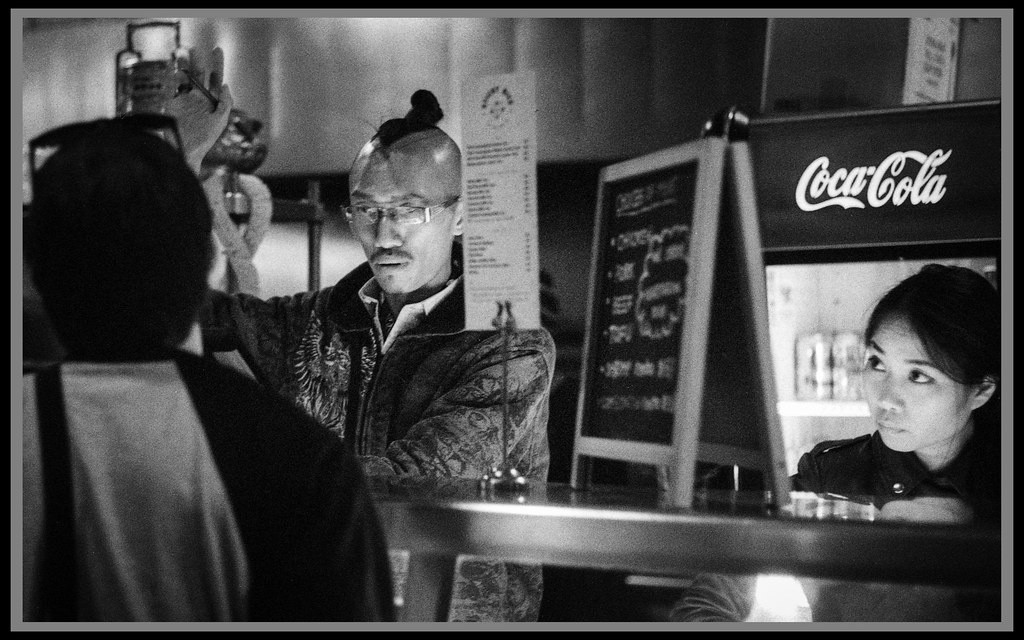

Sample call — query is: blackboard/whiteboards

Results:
[575,135,787,467]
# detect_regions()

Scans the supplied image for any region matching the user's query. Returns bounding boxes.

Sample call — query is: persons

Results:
[164,47,755,622]
[21,84,394,619]
[790,263,998,496]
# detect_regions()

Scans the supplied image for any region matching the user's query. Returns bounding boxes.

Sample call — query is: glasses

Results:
[22,106,184,185]
[335,198,461,230]
